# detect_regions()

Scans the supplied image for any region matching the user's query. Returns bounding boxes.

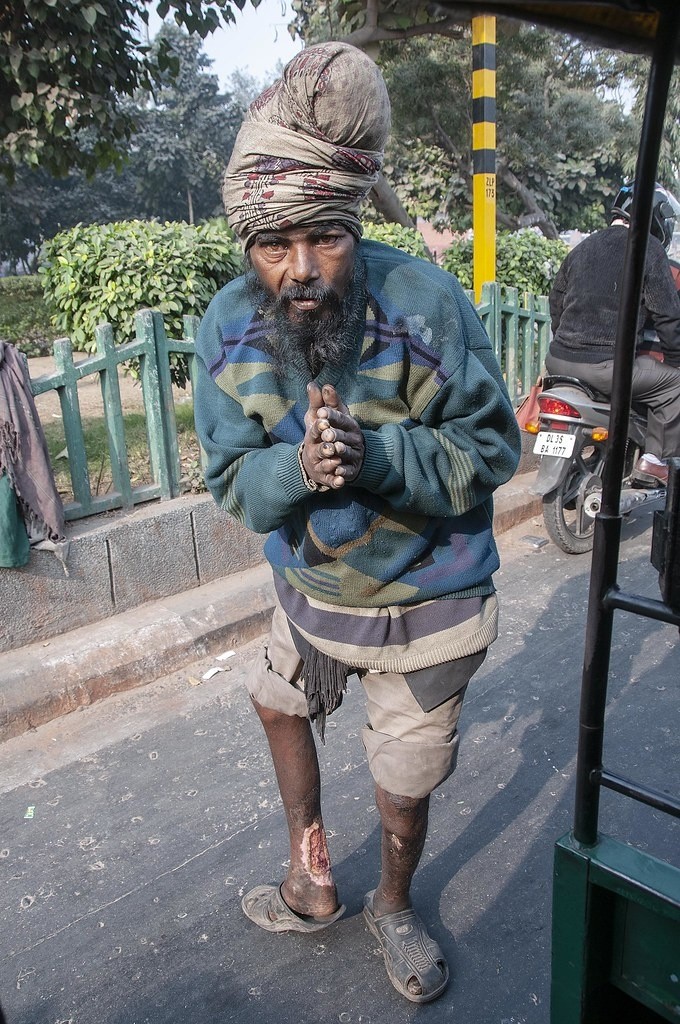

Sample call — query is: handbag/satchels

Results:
[514,374,545,435]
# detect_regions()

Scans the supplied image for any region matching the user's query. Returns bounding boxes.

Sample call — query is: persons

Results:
[544,179,680,488]
[192,43,524,1005]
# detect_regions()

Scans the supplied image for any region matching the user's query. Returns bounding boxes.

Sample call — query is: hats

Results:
[217,40,422,240]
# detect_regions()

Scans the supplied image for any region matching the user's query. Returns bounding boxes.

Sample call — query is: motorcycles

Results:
[523,251,679,555]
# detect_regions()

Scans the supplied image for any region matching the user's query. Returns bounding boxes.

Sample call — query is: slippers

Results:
[241,880,347,932]
[363,885,449,1003]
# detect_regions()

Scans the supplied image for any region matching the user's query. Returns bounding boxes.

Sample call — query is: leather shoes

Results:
[633,457,669,486]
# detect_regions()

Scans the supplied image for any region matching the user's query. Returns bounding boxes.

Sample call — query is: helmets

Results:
[609,179,676,254]
